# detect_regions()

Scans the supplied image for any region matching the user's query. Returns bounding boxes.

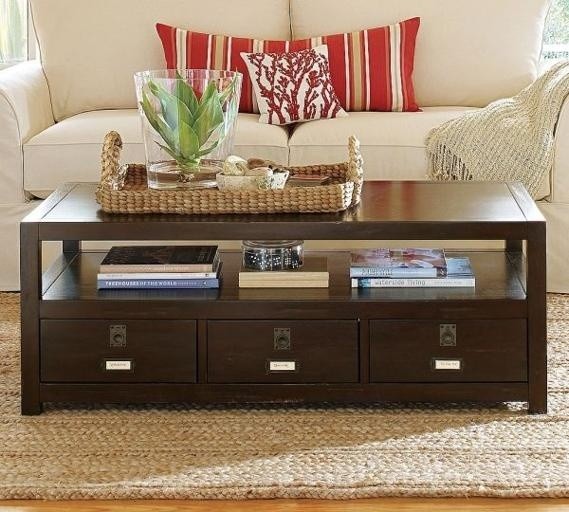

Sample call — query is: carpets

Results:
[3,292,568,499]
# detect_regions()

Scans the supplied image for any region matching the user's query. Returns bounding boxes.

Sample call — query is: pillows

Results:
[156,17,423,125]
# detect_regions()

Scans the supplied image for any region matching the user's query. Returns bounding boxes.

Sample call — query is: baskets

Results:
[95,131,364,215]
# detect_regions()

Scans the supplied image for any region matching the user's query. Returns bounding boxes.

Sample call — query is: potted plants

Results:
[134,69,244,190]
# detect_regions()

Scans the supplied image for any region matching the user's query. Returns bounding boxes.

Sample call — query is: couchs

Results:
[0,0,568,292]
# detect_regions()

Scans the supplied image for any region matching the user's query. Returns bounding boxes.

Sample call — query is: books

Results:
[350,248,476,288]
[97,246,223,289]
[239,257,329,288]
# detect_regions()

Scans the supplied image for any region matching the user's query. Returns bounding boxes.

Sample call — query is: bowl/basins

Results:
[214,168,291,189]
